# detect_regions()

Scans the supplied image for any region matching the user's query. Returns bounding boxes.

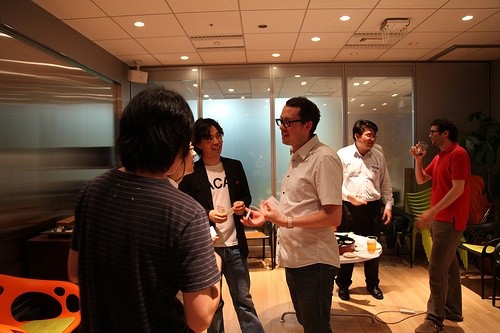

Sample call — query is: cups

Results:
[366,236,377,253]
[414,140,428,156]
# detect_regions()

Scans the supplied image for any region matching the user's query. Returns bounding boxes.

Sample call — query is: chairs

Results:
[381,187,500,306]
[245,205,274,270]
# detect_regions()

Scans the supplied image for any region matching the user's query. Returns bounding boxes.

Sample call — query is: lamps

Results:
[380,20,410,44]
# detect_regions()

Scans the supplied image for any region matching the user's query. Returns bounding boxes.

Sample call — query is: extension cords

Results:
[400,309,416,314]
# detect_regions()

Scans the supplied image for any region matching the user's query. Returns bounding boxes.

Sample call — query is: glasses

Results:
[176,145,194,157]
[430,130,440,134]
[364,134,377,138]
[275,118,303,129]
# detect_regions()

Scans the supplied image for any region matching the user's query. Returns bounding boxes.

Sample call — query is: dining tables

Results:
[280,232,382,325]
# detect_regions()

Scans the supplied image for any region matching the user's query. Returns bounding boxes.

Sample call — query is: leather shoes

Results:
[338,288,351,301]
[366,285,384,300]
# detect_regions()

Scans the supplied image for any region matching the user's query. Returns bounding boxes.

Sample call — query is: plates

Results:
[342,252,357,257]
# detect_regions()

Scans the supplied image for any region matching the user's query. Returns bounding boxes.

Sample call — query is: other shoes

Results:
[415,319,442,333]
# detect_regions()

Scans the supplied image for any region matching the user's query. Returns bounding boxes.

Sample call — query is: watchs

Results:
[286,216,293,229]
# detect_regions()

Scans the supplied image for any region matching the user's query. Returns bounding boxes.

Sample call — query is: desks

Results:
[26,234,73,278]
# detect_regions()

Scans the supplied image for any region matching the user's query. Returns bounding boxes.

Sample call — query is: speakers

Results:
[127,70,148,83]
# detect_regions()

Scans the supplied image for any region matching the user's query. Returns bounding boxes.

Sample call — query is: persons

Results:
[336,118,394,301]
[411,117,471,333]
[186,114,266,333]
[66,85,226,333]
[239,94,343,333]
